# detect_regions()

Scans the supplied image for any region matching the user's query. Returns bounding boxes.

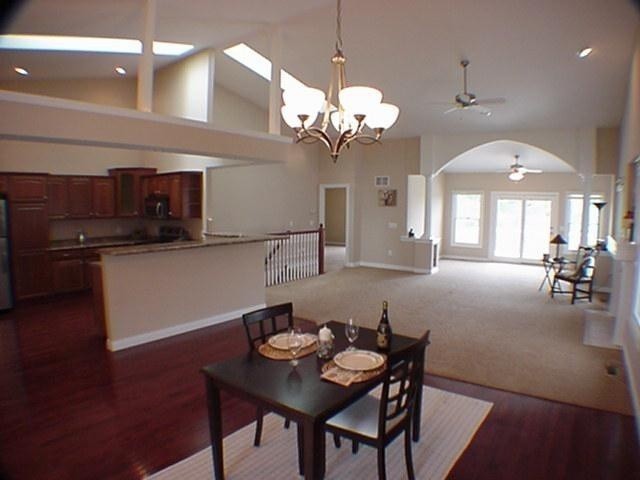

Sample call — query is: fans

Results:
[432,60,506,117]
[497,154,543,173]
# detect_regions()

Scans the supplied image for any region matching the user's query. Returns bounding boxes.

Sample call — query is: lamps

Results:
[281,0,401,164]
[550,234,568,261]
[508,173,524,181]
[591,202,607,244]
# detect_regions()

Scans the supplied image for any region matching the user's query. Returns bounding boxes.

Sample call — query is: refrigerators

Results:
[0,194,13,315]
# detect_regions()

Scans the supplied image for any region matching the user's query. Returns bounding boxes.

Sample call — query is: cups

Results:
[543,254,550,261]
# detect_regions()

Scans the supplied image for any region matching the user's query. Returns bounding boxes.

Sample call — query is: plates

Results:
[268,332,314,351]
[333,349,385,371]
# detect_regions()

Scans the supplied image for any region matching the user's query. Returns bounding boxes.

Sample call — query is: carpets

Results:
[143,376,494,480]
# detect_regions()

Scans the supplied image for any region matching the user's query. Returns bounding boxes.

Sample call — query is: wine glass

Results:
[345,317,360,352]
[287,326,304,367]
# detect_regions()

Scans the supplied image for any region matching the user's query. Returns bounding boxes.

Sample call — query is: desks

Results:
[538,257,576,298]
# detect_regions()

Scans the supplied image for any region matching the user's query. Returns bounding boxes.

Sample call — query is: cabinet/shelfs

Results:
[168,171,202,219]
[48,174,116,218]
[50,244,100,295]
[111,168,141,218]
[8,171,50,302]
[141,174,168,197]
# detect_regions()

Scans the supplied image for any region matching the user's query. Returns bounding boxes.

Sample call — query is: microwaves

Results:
[144,194,170,221]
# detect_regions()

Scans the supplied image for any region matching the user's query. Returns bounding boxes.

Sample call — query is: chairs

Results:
[550,247,600,304]
[243,302,294,447]
[324,329,430,480]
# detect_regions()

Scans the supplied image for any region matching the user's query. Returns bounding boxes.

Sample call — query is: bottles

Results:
[376,301,392,352]
[315,324,335,360]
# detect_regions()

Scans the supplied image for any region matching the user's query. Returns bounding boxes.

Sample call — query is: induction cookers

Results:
[154,226,186,243]
